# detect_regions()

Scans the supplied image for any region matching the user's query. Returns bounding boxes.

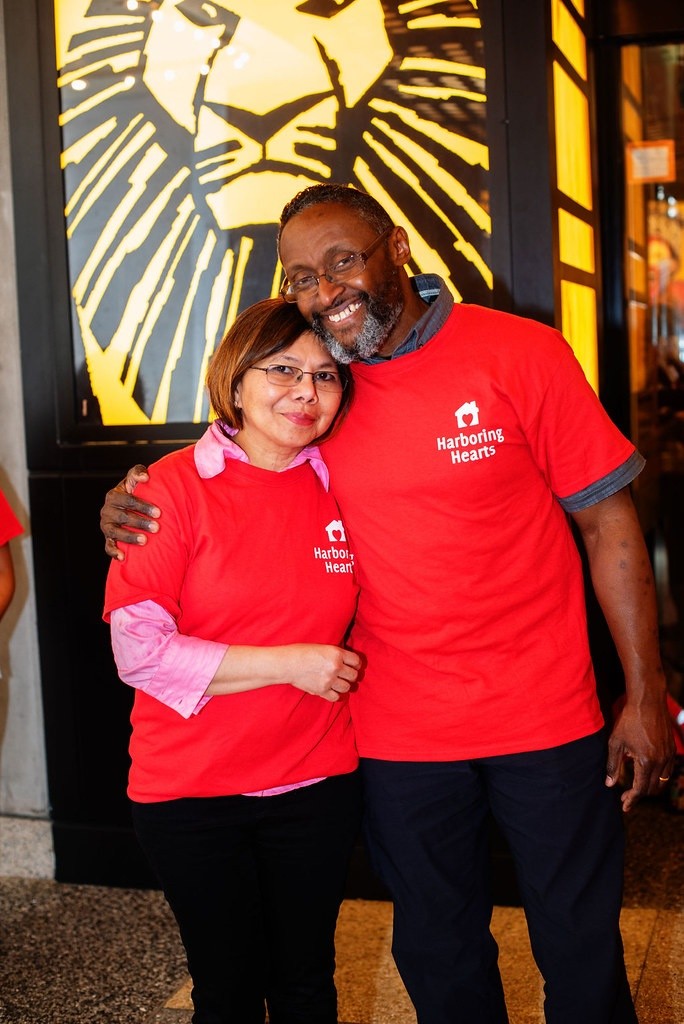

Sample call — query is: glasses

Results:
[279,226,394,303]
[249,361,348,393]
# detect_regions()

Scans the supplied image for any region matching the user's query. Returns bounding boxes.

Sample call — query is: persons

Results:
[102,297,364,1024]
[639,335,684,418]
[100,185,677,1023]
[0,489,25,619]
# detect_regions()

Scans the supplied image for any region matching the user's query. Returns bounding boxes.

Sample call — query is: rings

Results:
[659,775,670,781]
